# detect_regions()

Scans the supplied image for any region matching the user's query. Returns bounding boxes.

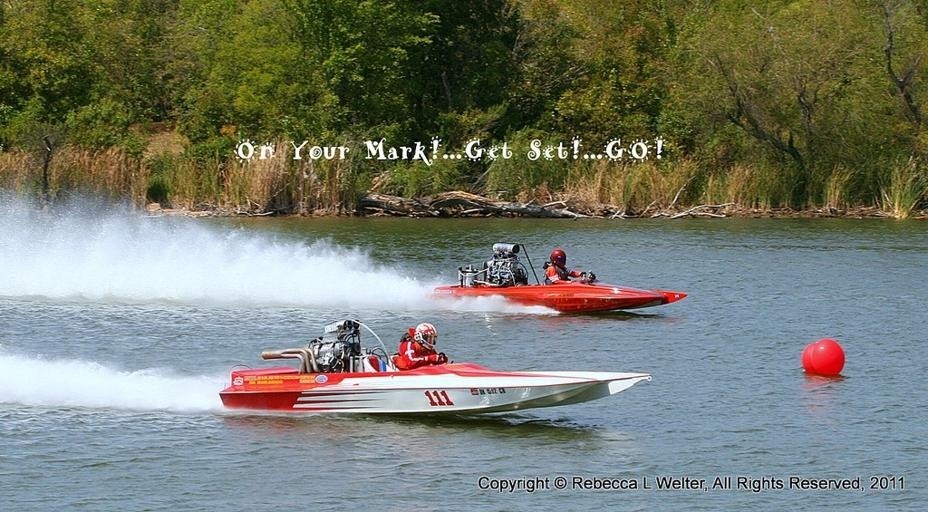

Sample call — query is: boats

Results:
[218,319,652,416]
[433,241,688,314]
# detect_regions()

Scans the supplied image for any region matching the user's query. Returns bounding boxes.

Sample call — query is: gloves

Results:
[428,353,449,363]
[579,270,596,283]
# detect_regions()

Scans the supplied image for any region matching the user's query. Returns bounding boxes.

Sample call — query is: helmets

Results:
[413,322,438,350]
[550,249,566,266]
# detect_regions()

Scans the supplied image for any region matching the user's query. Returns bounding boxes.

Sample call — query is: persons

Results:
[391,321,450,372]
[544,248,584,287]
